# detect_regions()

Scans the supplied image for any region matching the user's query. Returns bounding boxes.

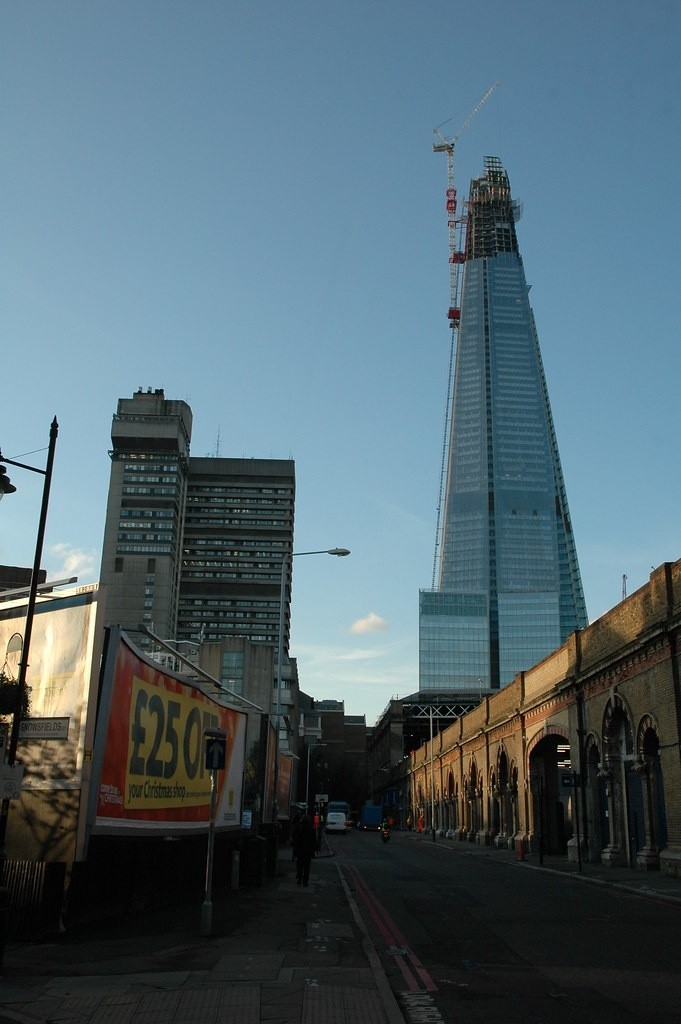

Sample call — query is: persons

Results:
[381,818,390,840]
[292,815,317,887]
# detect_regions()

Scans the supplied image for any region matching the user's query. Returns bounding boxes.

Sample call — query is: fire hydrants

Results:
[517,841,527,862]
[430,828,436,842]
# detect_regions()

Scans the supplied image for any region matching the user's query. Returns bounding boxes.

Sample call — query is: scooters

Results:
[379,824,390,843]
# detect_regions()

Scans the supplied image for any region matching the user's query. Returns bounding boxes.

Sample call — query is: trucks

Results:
[362,805,383,832]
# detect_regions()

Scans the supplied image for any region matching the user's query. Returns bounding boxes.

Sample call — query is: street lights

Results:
[270,546,352,730]
[0,415,61,875]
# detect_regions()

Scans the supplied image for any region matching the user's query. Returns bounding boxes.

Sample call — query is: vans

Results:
[326,812,346,834]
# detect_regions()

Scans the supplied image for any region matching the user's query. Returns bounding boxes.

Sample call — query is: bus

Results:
[328,801,353,831]
